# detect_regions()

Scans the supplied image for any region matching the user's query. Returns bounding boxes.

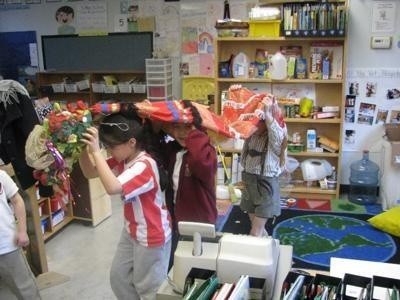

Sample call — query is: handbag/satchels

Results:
[216,204,251,235]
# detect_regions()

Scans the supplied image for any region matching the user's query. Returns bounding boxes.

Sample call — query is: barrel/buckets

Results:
[349,150,380,205]
[233,50,249,78]
[301,158,336,183]
[269,51,287,81]
[280,156,301,177]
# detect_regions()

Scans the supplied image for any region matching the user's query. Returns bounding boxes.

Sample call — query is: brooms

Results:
[218,146,239,204]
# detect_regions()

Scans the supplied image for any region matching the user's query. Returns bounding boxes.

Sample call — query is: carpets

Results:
[218,203,400,272]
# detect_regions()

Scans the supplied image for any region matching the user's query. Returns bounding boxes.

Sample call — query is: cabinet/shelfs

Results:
[0,153,113,276]
[214,2,349,200]
[36,71,148,105]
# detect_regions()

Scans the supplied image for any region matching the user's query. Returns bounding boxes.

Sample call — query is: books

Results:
[182,271,399,300]
[283,4,347,37]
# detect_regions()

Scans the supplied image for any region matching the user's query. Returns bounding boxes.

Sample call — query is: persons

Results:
[0,169,41,300]
[142,98,219,274]
[230,82,287,237]
[126,4,139,24]
[77,110,175,300]
